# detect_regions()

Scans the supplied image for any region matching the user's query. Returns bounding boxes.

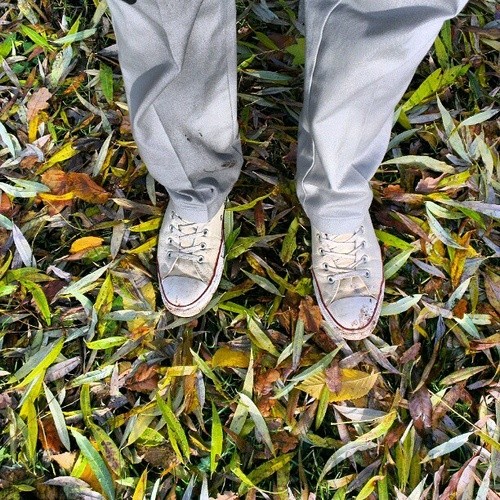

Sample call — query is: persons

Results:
[101,0,473,341]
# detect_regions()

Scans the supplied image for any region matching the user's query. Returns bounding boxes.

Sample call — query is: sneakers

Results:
[312,216,386,339]
[156,200,224,318]
